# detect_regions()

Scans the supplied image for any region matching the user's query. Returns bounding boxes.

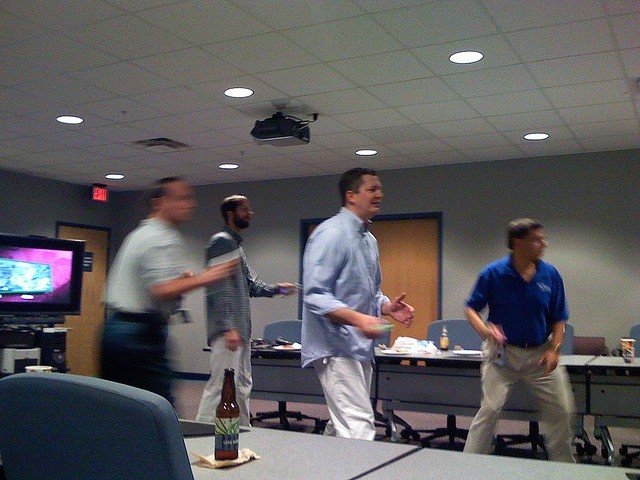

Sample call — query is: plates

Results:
[380,349,413,355]
[273,345,303,351]
[452,350,484,356]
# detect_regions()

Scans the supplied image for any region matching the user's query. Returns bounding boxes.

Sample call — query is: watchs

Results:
[550,341,564,356]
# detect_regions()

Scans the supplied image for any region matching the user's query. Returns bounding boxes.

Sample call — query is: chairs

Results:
[399,318,488,450]
[316,319,421,442]
[250,320,321,430]
[619,323,640,467]
[0,371,195,480]
[574,336,606,355]
[495,322,575,458]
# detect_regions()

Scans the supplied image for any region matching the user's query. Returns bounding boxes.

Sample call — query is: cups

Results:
[620,340,636,367]
[25,366,52,372]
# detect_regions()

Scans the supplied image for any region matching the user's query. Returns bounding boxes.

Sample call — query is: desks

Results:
[201,338,375,409]
[352,447,636,480]
[585,353,640,465]
[180,427,422,480]
[373,348,597,464]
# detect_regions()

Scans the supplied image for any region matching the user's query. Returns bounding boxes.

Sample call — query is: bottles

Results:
[215,367,239,460]
[439,323,451,350]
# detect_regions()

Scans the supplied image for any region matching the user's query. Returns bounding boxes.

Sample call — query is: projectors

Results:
[248,113,310,148]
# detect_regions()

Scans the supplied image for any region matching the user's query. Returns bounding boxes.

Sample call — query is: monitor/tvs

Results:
[0,233,88,324]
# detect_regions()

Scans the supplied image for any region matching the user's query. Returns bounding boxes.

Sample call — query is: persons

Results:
[459,217,579,464]
[300,167,414,442]
[94,177,237,407]
[195,191,297,428]
